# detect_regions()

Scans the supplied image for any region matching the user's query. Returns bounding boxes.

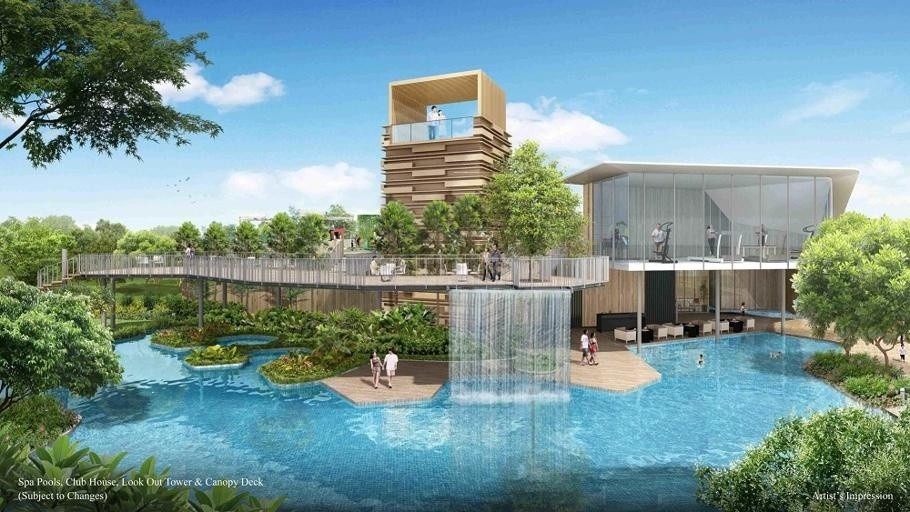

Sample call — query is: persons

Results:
[588,332,599,366]
[899,344,906,359]
[652,224,665,259]
[370,350,382,389]
[706,226,715,255]
[482,244,506,281]
[184,244,195,267]
[370,256,385,281]
[427,106,446,139]
[579,330,592,365]
[387,257,404,281]
[757,224,766,247]
[698,354,704,367]
[739,301,745,311]
[382,349,398,389]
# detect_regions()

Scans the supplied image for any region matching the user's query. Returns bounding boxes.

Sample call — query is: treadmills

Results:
[687,232,746,262]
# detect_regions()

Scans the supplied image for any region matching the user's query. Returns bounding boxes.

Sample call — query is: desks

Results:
[743,244,776,258]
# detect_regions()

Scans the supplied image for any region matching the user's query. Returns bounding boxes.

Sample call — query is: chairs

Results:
[443,263,481,278]
[596,311,756,345]
[370,258,406,277]
